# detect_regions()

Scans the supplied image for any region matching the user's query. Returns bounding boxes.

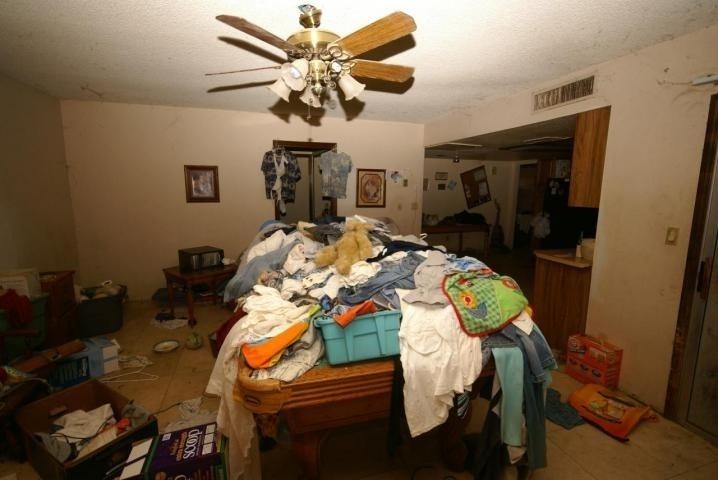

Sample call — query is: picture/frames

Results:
[355,167,387,208]
[183,164,221,204]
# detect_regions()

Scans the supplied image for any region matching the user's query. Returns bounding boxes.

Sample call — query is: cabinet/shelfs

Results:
[530,259,593,353]
[568,107,612,207]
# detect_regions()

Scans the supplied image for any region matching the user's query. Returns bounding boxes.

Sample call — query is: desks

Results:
[162,264,238,329]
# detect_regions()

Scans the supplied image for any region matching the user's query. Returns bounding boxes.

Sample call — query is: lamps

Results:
[265,56,368,111]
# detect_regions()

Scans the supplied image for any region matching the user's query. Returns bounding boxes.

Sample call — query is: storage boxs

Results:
[318,309,403,367]
[2,338,231,480]
[68,285,130,339]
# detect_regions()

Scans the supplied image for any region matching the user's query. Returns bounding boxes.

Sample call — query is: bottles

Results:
[576,239,583,259]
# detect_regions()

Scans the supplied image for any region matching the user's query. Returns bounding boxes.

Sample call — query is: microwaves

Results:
[179,246,224,272]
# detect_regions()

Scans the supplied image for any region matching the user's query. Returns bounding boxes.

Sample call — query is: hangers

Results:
[391,233,429,246]
[320,144,351,158]
[265,143,297,158]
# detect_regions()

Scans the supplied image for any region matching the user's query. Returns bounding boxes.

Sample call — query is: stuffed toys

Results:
[315,221,376,275]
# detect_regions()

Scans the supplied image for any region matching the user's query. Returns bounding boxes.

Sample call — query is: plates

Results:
[152,340,179,352]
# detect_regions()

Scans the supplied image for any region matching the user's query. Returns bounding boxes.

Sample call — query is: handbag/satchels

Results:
[0,368,54,462]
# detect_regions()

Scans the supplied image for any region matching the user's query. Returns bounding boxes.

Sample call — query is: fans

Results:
[203,3,418,84]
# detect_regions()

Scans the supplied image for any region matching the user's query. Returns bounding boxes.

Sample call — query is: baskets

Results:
[38,269,61,293]
